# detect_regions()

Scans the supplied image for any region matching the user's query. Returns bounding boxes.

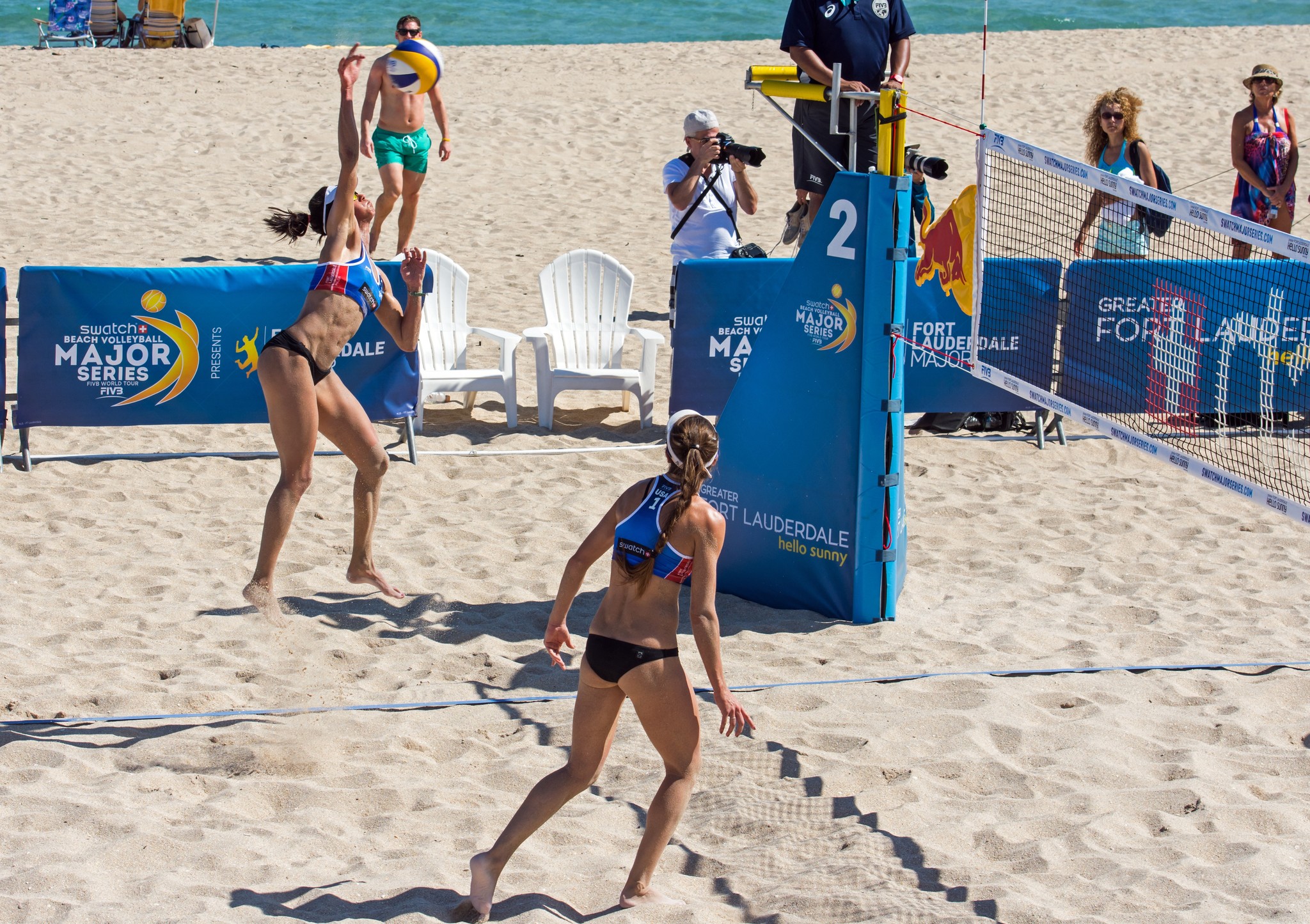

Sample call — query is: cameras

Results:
[705,132,766,167]
[732,243,768,259]
[904,148,948,180]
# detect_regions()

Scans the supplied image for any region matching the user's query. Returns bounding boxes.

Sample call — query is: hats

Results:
[666,409,719,470]
[323,175,359,234]
[683,109,719,142]
[1242,64,1283,92]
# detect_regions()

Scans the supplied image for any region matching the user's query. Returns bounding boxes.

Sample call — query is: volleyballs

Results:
[385,38,445,95]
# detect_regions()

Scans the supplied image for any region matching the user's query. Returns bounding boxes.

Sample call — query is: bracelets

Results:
[407,290,426,296]
[442,138,449,142]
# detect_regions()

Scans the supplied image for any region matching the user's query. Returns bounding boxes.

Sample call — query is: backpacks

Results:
[965,408,1027,431]
[1129,139,1174,239]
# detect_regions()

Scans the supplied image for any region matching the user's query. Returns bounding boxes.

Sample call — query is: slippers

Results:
[261,43,280,48]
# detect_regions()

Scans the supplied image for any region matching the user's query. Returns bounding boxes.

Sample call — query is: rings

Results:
[729,717,736,719]
[1278,200,1281,203]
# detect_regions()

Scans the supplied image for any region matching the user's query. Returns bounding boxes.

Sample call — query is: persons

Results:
[1229,65,1299,259]
[96,0,146,48]
[468,410,758,924]
[242,45,427,627]
[360,14,451,256]
[663,110,758,372]
[1074,87,1157,261]
[779,1,936,259]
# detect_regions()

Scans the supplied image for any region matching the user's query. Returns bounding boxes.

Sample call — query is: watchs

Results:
[888,74,904,85]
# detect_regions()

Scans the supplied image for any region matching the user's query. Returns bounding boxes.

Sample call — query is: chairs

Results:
[523,250,665,429]
[392,249,522,431]
[33,0,186,49]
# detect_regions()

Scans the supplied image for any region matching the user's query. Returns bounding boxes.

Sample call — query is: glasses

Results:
[688,137,710,147]
[1250,77,1278,86]
[352,191,358,201]
[1102,112,1124,119]
[397,28,420,37]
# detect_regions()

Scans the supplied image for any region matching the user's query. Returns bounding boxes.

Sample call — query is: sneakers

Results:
[783,198,811,249]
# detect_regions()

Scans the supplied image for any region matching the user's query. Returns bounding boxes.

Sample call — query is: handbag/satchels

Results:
[909,412,970,432]
[183,17,214,49]
